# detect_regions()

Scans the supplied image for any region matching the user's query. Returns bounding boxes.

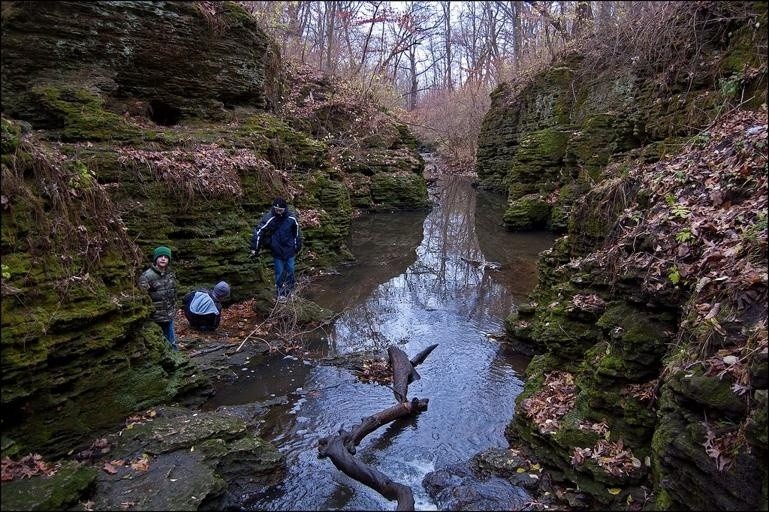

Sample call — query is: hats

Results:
[212,281,231,300]
[153,246,171,262]
[273,196,287,209]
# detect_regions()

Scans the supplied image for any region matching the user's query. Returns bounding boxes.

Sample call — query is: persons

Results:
[182,280,231,332]
[139,245,179,353]
[249,197,302,304]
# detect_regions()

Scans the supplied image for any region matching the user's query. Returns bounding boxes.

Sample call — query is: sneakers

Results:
[277,293,297,304]
